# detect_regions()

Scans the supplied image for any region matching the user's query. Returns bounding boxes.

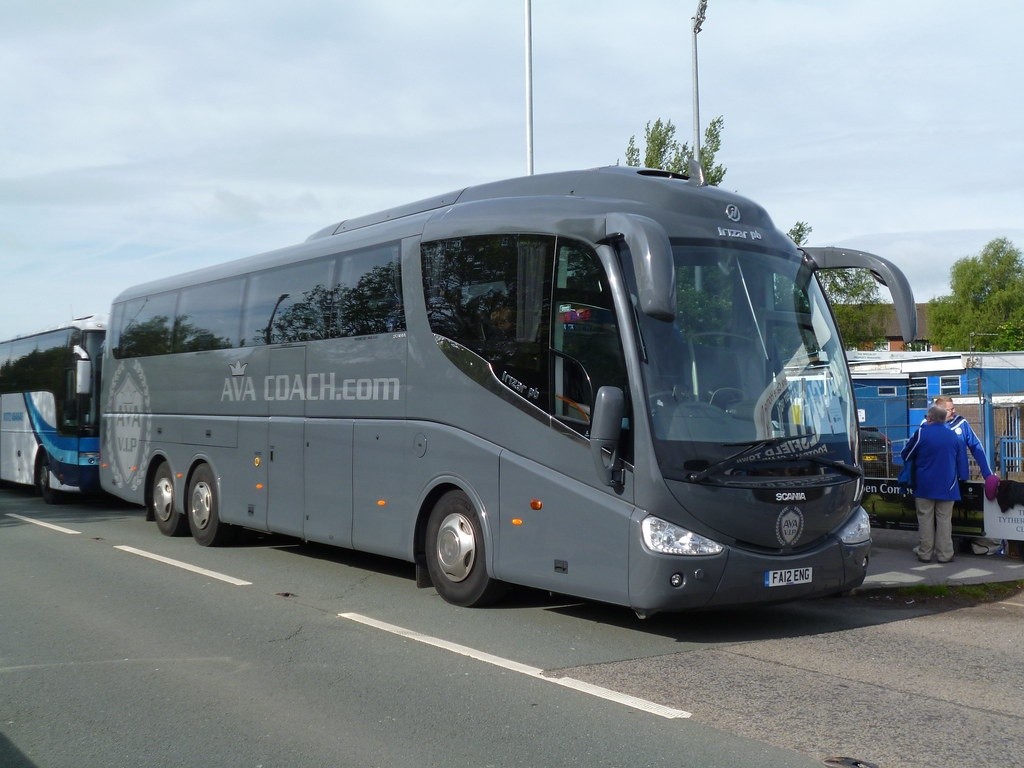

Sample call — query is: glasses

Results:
[925,415,930,419]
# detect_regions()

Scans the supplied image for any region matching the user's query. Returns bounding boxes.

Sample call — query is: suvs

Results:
[858,428,893,479]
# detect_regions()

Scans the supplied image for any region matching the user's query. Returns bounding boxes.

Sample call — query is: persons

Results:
[489,306,517,367]
[901,396,993,563]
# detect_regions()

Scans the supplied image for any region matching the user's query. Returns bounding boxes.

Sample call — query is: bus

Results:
[0,313,110,504]
[98,164,918,621]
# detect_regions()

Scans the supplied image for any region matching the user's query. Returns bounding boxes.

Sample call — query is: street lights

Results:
[690,0,707,161]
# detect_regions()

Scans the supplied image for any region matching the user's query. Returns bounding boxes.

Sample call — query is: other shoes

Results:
[919,559,930,563]
